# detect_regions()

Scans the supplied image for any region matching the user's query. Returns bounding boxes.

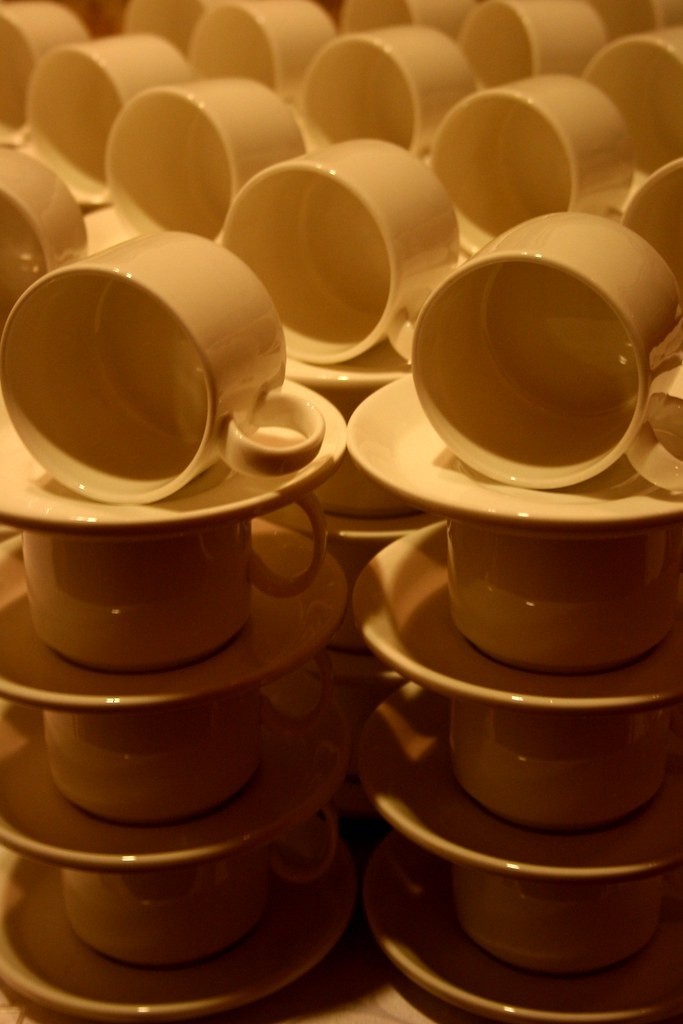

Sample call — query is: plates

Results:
[284,343,419,388]
[0,695,353,864]
[16,121,107,204]
[347,380,683,527]
[354,518,683,708]
[0,849,355,1020]
[0,518,348,709]
[361,830,683,1021]
[87,204,158,253]
[0,376,349,525]
[357,682,683,875]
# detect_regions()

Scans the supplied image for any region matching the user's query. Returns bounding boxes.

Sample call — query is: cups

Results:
[451,863,667,977]
[410,213,683,499]
[42,684,265,821]
[27,34,188,204]
[192,0,334,82]
[580,27,683,168]
[223,138,461,364]
[340,0,481,27]
[0,0,91,145]
[60,807,337,964]
[107,79,304,238]
[429,77,632,253]
[0,229,328,504]
[122,0,205,56]
[0,149,86,270]
[450,697,672,829]
[447,524,683,672]
[306,28,472,155]
[21,494,329,671]
[465,1,607,88]
